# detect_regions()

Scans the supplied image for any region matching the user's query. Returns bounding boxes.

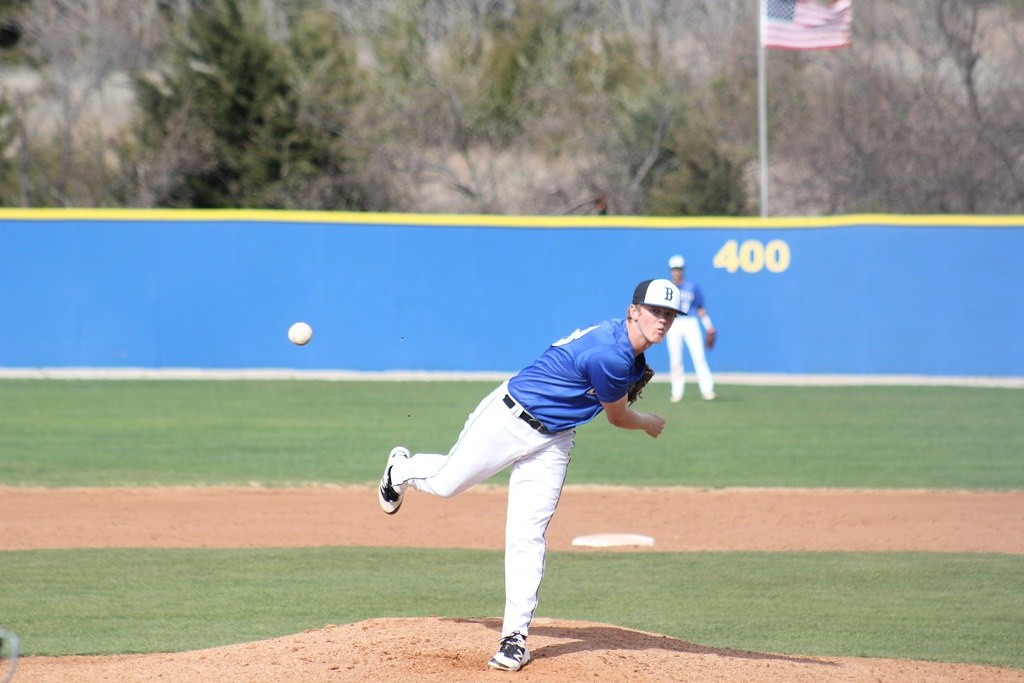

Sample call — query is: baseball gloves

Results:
[627,363,655,403]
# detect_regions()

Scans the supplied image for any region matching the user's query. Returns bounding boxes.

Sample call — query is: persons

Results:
[663,255,717,402]
[378,280,690,670]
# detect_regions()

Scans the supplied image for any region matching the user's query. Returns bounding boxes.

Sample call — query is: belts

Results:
[502,394,553,434]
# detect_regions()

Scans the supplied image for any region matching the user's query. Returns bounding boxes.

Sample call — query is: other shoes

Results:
[703,392,716,400]
[671,395,682,403]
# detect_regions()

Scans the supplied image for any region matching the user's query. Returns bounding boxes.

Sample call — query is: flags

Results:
[762,0,853,47]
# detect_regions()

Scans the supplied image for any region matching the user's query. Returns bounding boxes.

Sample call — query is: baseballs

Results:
[287,322,313,346]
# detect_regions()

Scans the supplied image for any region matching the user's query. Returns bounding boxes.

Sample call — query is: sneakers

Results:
[378,446,410,514]
[488,630,531,671]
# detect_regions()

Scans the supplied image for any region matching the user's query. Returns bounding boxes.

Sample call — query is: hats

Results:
[632,278,688,316]
[668,255,685,269]
